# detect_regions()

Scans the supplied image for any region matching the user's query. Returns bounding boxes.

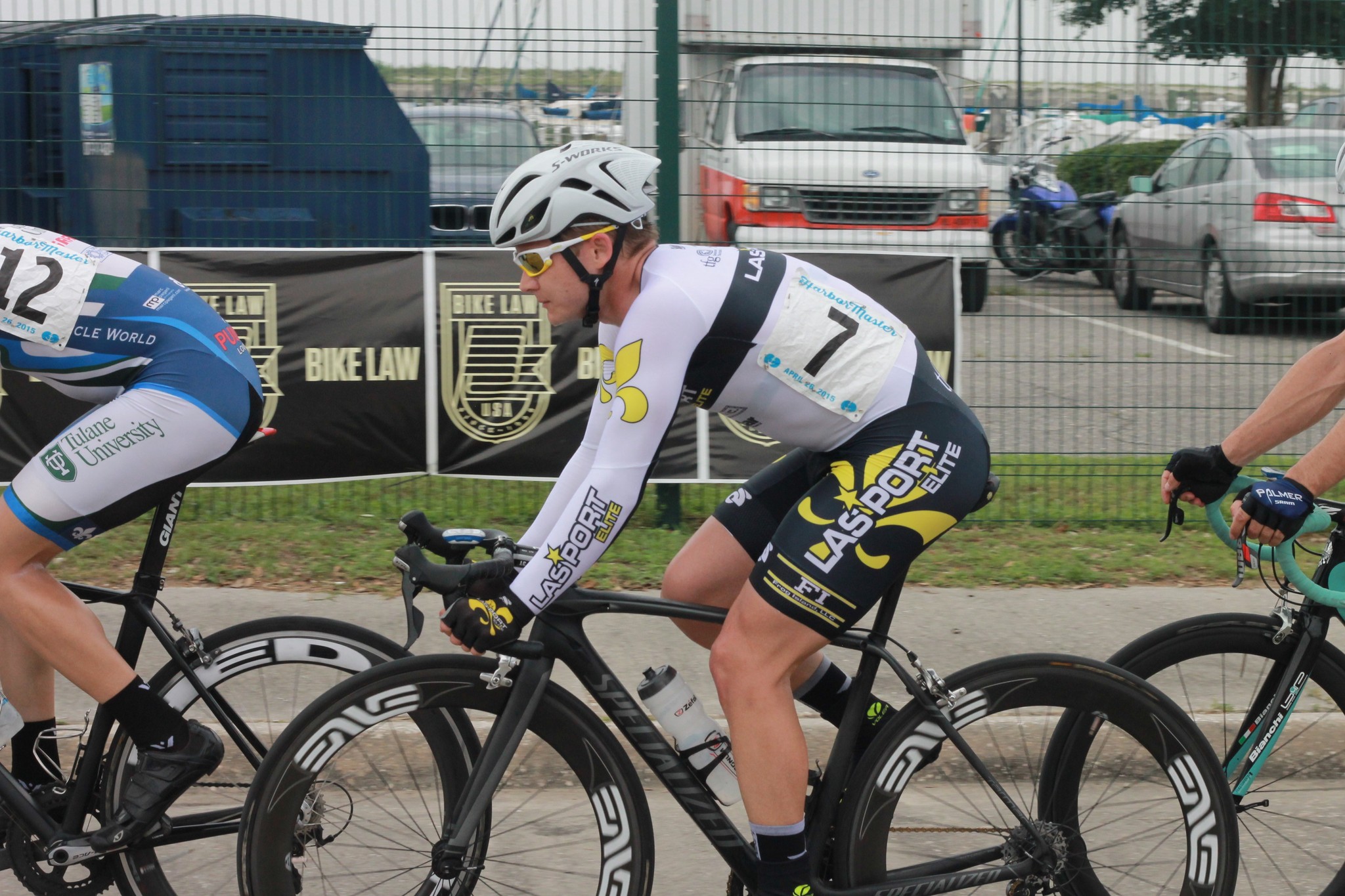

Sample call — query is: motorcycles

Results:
[990,164,1120,290]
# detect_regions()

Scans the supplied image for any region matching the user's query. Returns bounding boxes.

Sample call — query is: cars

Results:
[1290,94,1345,129]
[397,105,548,249]
[1108,126,1345,336]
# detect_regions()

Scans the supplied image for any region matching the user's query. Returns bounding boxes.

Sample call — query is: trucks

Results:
[674,0,993,314]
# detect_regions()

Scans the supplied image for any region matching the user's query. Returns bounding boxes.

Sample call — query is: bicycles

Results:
[0,424,492,896]
[1035,469,1344,896]
[235,474,1239,896]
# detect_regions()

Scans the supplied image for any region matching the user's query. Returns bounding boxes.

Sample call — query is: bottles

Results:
[636,663,745,806]
[0,686,24,745]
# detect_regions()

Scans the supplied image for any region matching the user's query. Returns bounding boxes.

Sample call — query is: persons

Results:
[1162,331,1345,543]
[438,142,991,896]
[2,223,262,868]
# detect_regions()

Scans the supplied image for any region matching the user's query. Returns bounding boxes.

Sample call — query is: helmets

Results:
[490,139,661,247]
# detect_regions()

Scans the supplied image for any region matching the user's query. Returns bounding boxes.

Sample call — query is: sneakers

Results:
[91,719,224,852]
[0,779,68,812]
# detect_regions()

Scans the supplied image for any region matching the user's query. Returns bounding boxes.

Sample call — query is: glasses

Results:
[513,225,619,276]
